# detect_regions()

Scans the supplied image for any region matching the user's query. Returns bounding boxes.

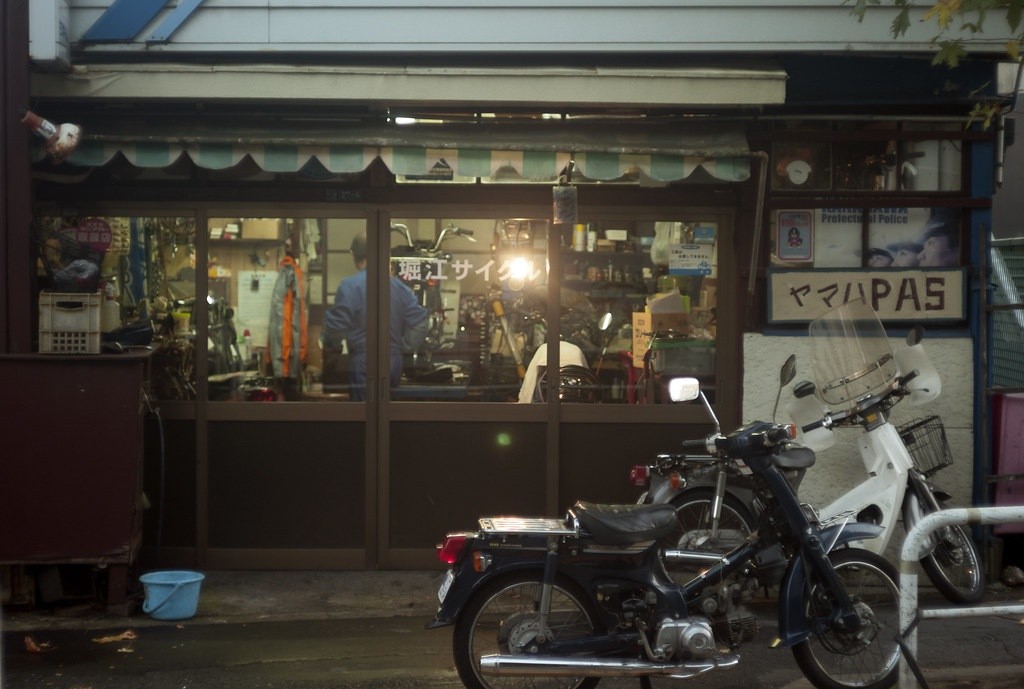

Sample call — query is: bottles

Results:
[243,330,253,360]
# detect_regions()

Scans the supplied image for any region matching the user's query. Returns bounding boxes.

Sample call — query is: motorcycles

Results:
[425,353,904,689]
[393,220,479,344]
[631,292,985,622]
[163,287,248,402]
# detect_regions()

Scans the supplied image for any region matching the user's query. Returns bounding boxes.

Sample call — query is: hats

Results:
[351,232,366,255]
[870,214,959,255]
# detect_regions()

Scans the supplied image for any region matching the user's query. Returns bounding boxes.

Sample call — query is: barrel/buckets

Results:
[140,570,205,619]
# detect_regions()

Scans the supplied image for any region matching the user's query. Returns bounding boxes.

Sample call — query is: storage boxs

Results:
[38,256,101,354]
[632,288,717,368]
[646,338,717,377]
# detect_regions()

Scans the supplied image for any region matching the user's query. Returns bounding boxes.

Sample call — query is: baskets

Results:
[896,415,953,476]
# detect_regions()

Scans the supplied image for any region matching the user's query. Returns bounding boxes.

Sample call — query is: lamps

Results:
[17,109,81,168]
[507,257,534,281]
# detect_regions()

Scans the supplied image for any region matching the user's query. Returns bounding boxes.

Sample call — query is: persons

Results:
[886,242,924,267]
[915,224,960,266]
[853,246,894,267]
[322,232,428,403]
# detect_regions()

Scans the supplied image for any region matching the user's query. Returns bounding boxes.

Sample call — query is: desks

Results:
[0,344,164,618]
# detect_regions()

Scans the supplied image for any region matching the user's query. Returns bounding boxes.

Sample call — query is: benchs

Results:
[298,375,521,403]
